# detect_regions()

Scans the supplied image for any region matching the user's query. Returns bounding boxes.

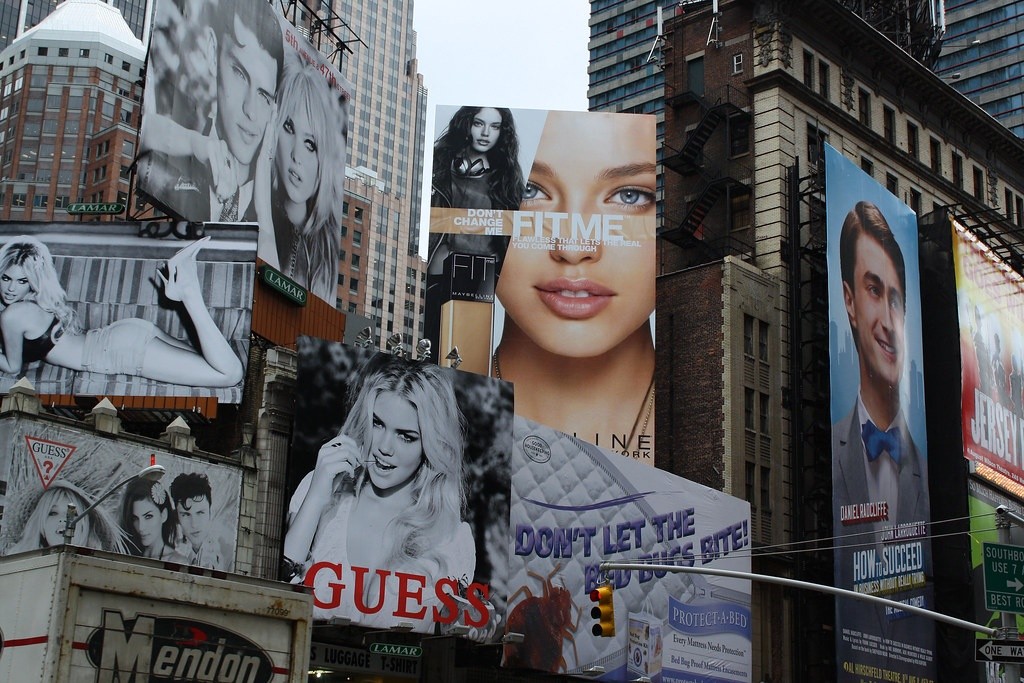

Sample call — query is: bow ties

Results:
[861,419,902,466]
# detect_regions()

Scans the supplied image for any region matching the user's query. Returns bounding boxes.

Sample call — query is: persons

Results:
[116,475,191,565]
[138,0,348,311]
[489,111,656,468]
[282,350,477,635]
[424,106,527,368]
[0,235,242,388]
[832,199,932,683]
[972,306,1024,419]
[5,478,142,556]
[170,472,233,572]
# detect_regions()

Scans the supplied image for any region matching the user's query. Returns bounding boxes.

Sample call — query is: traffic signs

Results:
[975,639,1024,664]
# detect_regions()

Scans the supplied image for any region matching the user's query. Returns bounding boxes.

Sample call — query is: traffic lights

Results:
[590,583,615,638]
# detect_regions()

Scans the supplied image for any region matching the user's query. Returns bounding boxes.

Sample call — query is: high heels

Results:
[156,237,212,302]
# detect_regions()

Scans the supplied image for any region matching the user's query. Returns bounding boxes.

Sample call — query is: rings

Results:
[225,156,232,169]
[267,149,273,159]
[332,443,344,449]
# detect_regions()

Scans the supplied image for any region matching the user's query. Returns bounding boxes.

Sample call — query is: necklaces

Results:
[493,350,654,459]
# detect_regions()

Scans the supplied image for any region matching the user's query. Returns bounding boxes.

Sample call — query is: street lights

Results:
[65,465,166,544]
